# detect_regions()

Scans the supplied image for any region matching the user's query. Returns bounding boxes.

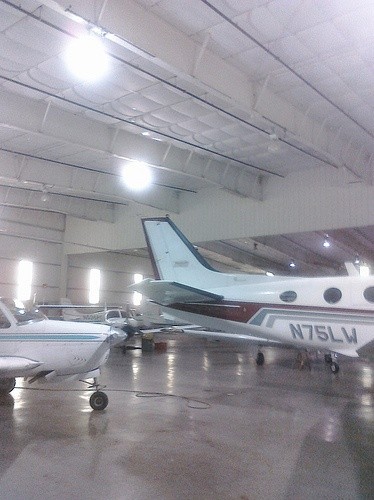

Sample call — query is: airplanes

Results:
[0,296,127,410]
[128,217,373,365]
[59,298,138,341]
